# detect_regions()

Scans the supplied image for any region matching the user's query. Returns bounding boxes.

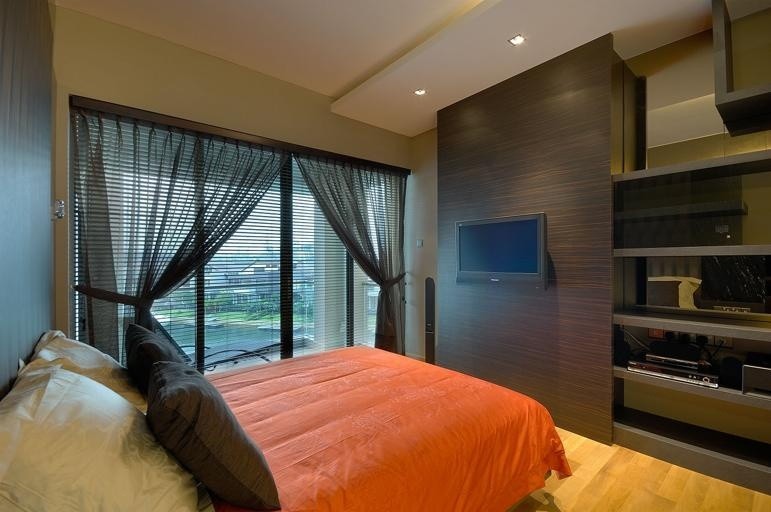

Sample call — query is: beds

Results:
[203,346,572,512]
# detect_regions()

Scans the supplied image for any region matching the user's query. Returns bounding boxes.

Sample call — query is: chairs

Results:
[150,314,281,372]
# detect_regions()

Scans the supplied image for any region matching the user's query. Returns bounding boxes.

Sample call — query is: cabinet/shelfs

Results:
[612,149,770,495]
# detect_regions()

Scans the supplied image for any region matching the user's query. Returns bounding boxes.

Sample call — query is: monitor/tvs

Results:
[456,212,547,291]
